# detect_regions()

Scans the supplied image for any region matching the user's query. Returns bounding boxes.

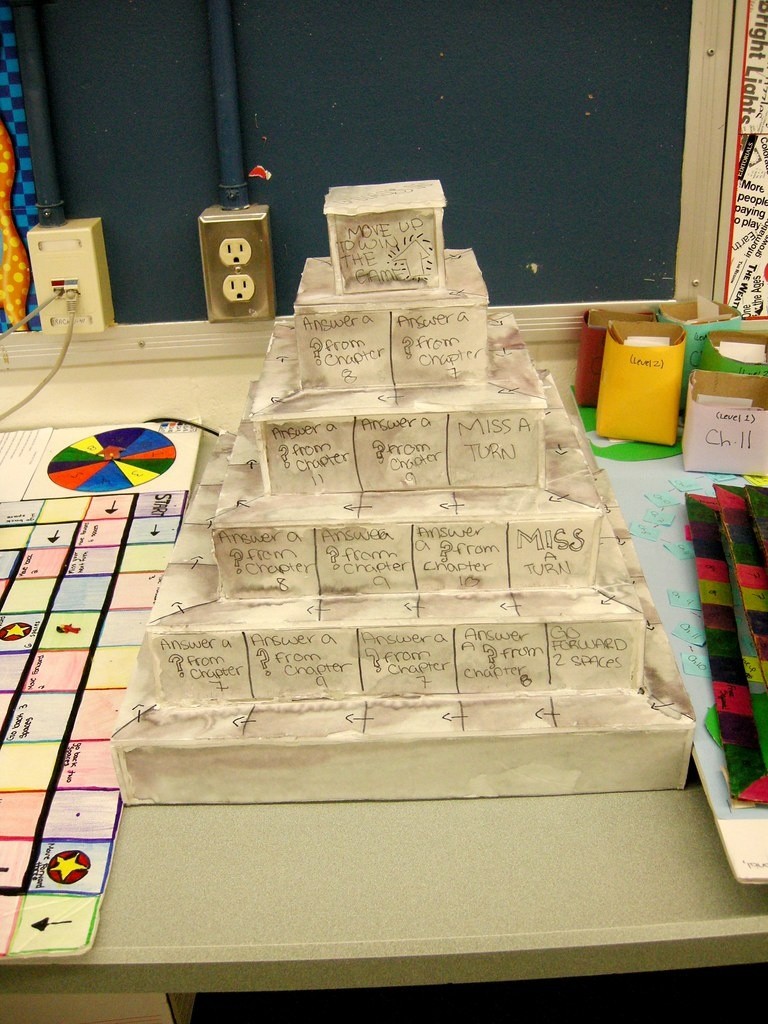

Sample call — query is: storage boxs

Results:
[248,312,550,498]
[324,179,448,296]
[654,299,741,417]
[294,248,490,393]
[109,467,697,808]
[681,369,768,476]
[210,369,605,601]
[696,329,768,378]
[575,309,661,409]
[596,319,687,447]
[144,426,648,711]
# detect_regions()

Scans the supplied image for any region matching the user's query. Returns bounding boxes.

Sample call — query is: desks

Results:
[0,413,768,993]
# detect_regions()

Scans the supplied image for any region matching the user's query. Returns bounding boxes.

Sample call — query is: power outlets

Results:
[197,203,276,324]
[26,217,115,335]
